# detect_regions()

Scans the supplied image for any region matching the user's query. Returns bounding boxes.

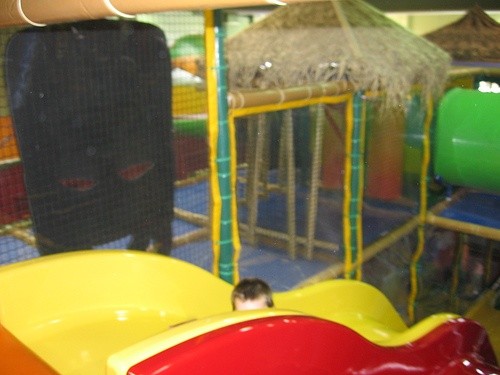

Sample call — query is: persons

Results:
[229,278,274,313]
